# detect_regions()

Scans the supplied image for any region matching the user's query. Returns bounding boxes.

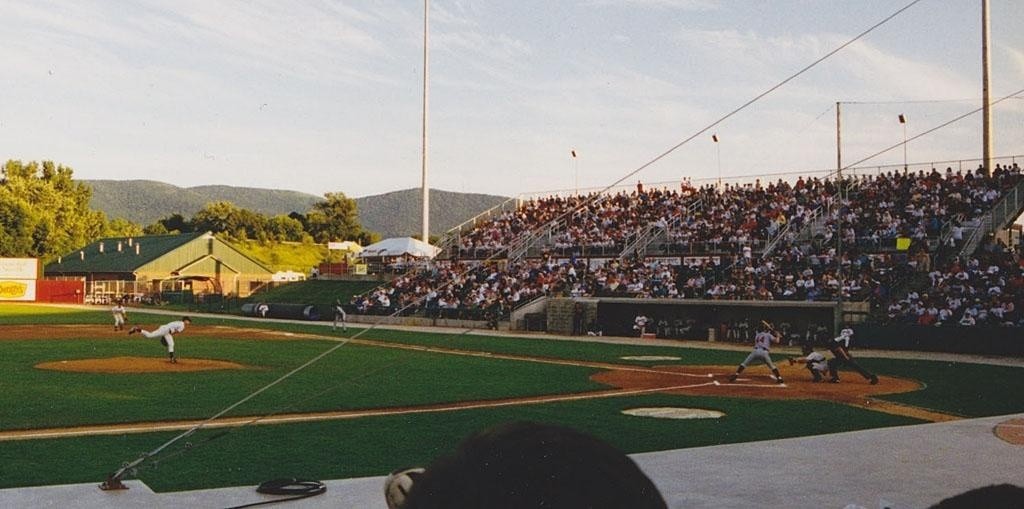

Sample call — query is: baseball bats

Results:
[762,319,783,338]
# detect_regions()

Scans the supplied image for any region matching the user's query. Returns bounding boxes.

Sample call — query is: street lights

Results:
[712,133,720,180]
[571,149,577,192]
[899,114,906,165]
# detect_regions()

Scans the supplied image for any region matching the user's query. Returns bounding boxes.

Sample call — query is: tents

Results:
[355,237,443,258]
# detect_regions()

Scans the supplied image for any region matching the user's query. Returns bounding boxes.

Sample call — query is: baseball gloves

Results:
[160,336,168,347]
[788,355,796,366]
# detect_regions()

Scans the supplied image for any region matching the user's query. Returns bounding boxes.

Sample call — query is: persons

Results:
[822,336,879,384]
[128,316,191,365]
[112,303,127,331]
[331,164,1024,347]
[728,325,784,384]
[787,343,828,382]
[401,422,668,509]
